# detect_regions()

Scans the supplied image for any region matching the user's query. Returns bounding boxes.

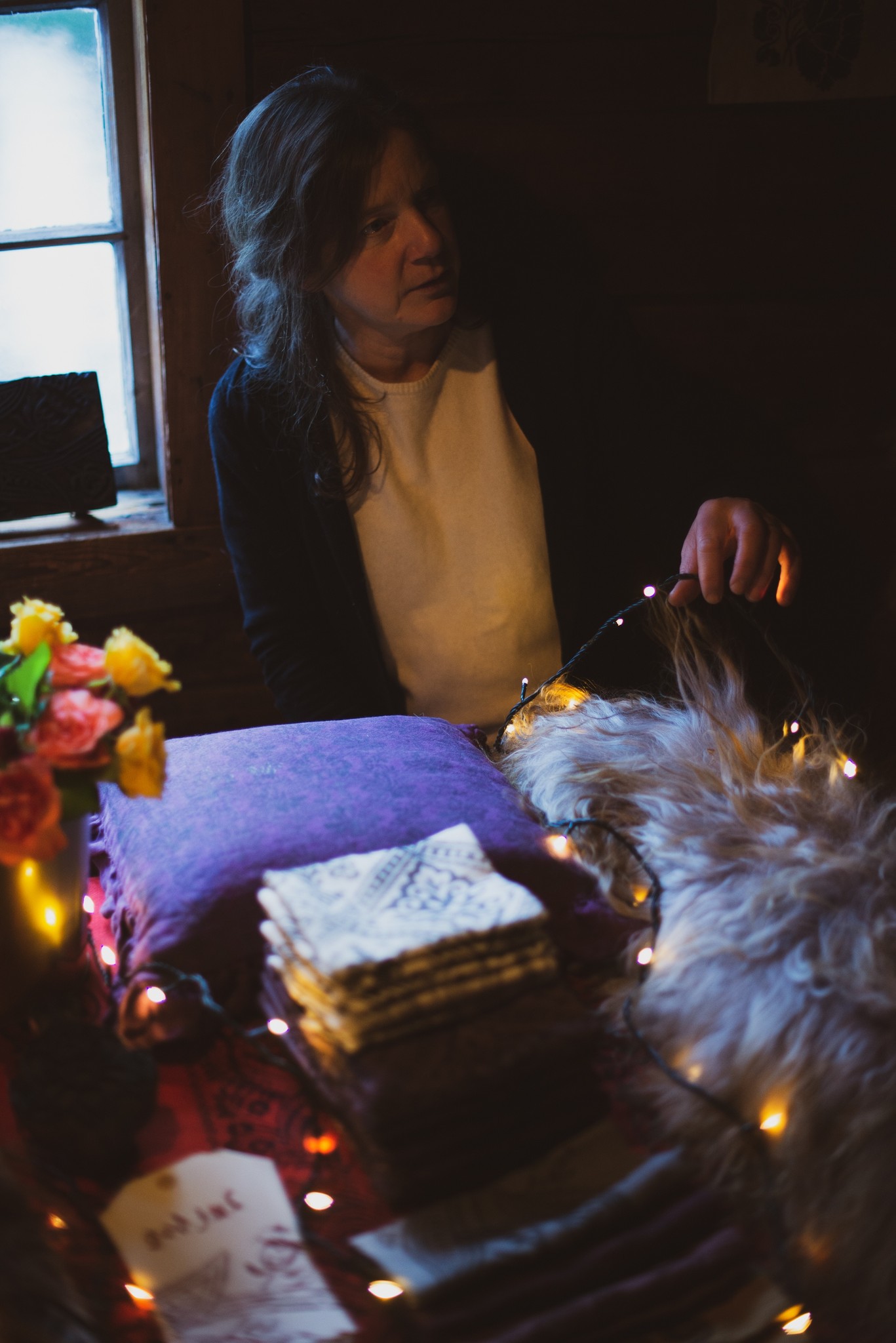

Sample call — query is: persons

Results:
[206,61,834,732]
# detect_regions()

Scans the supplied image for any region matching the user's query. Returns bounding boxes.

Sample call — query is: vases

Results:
[1,768,96,979]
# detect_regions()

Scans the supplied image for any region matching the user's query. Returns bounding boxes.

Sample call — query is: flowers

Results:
[1,596,184,866]
